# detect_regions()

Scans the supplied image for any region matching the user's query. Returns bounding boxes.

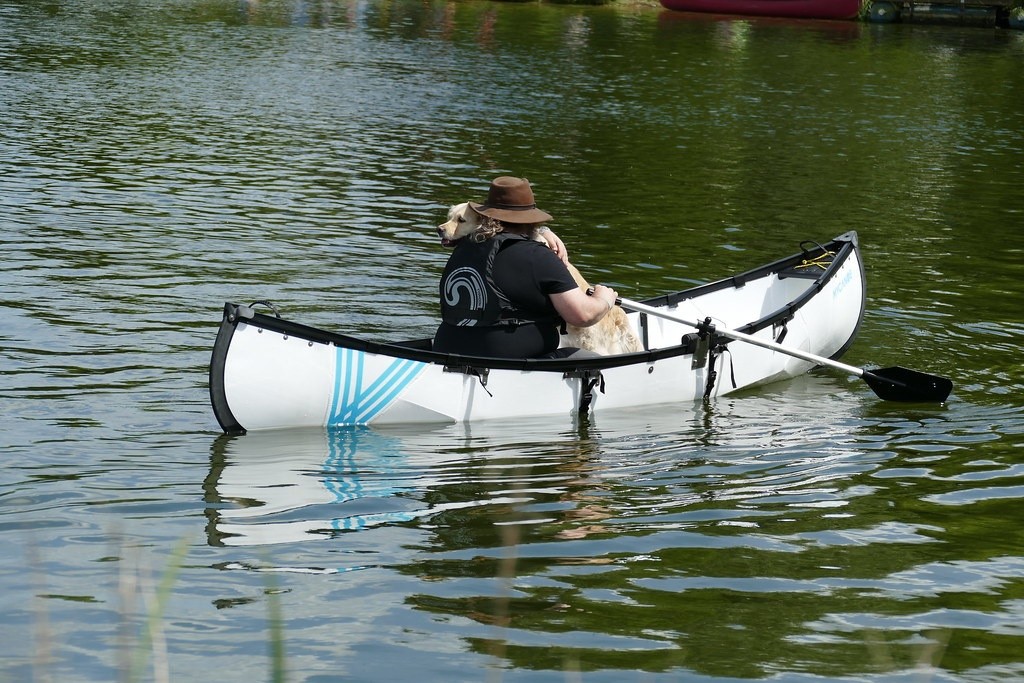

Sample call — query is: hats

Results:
[468,176,553,224]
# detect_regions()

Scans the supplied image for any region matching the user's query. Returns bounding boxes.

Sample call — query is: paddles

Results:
[582,284,954,404]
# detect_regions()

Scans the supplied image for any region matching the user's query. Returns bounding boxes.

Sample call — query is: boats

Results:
[209,229,868,437]
[659,0,1024,26]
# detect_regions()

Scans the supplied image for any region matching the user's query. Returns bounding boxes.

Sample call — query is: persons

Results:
[432,174,620,365]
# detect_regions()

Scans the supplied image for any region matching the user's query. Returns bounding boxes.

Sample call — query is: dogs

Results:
[437,201,643,356]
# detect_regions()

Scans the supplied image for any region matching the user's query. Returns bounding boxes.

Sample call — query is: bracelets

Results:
[591,294,613,312]
[538,226,551,235]
